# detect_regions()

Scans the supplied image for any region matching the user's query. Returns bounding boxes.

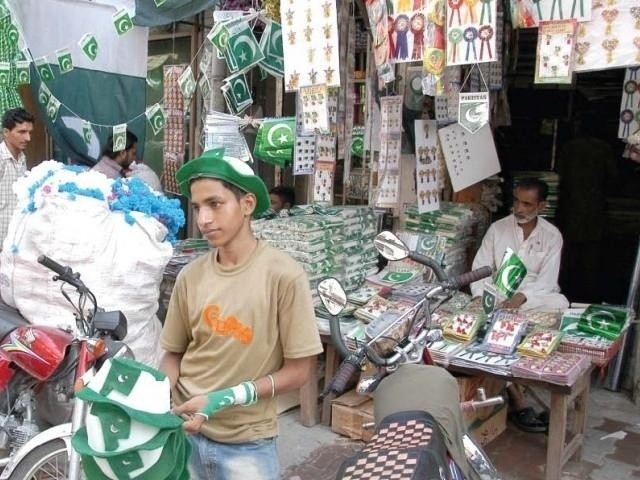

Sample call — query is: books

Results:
[453,375,483,429]
[467,403,507,447]
[330,389,375,442]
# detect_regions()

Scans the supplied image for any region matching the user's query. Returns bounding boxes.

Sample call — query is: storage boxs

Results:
[453,375,483,429]
[330,389,375,442]
[467,403,507,447]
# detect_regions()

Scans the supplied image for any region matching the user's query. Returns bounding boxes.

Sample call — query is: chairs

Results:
[508,407,547,432]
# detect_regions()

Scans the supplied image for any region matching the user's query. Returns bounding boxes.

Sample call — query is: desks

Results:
[299,328,598,479]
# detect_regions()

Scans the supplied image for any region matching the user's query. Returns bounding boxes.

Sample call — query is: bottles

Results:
[71,358,191,480]
[175,147,270,216]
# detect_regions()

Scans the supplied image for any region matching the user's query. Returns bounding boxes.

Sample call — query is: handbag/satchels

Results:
[269,374,276,399]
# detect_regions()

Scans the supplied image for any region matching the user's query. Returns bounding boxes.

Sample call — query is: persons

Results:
[469,178,571,310]
[1,108,35,252]
[270,184,296,212]
[90,130,138,179]
[157,150,324,480]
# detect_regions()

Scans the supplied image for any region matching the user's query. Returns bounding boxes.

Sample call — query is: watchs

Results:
[315,229,498,480]
[1,255,128,480]
